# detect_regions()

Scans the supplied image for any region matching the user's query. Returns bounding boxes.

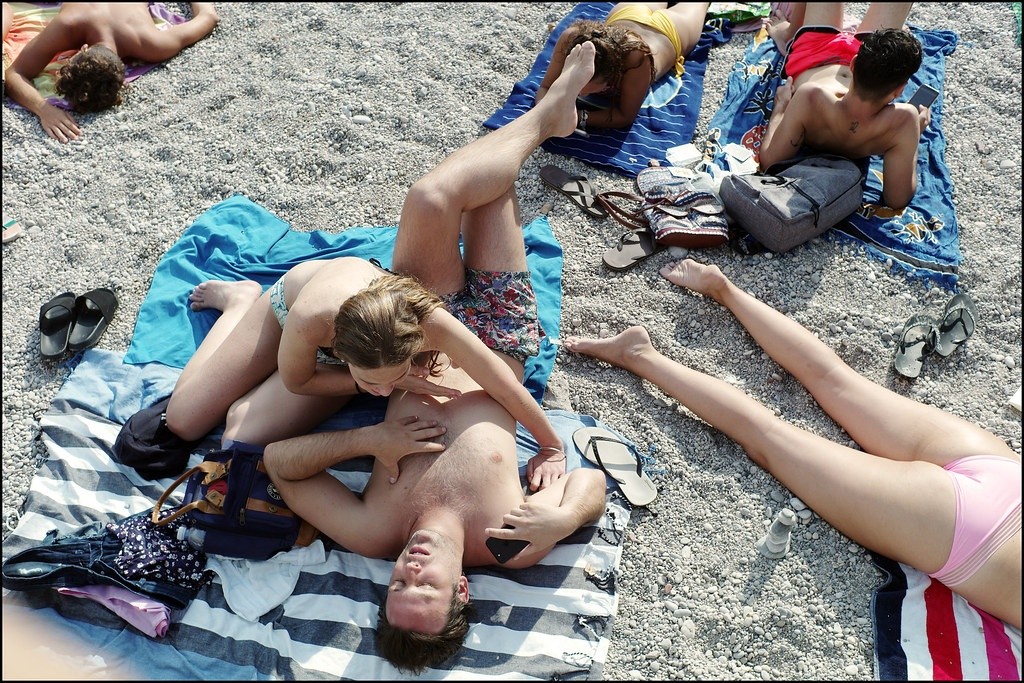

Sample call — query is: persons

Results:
[5,1,217,144]
[535,1,710,130]
[263,39,594,676]
[759,1,931,210]
[166,256,565,492]
[564,258,1022,630]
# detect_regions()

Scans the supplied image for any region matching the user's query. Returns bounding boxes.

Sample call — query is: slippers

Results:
[536,164,611,220]
[601,224,668,269]
[934,291,977,358]
[571,425,655,510]
[38,284,118,359]
[890,311,937,382]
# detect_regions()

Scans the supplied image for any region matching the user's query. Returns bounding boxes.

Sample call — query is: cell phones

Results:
[485,524,530,565]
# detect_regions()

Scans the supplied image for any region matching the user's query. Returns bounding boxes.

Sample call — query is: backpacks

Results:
[719,146,866,248]
[596,160,728,248]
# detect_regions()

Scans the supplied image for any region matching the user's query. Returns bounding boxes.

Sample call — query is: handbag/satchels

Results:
[150,438,323,557]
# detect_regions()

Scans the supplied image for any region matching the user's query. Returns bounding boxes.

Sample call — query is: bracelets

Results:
[539,447,560,452]
[579,109,588,128]
[355,381,363,394]
